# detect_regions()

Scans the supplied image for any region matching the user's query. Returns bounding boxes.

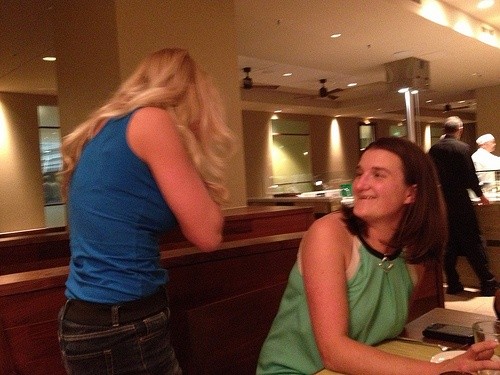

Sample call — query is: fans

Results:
[294,79,345,100]
[432,105,470,113]
[240,67,280,89]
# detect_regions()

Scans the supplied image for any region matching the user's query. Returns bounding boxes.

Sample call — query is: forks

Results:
[396,339,468,351]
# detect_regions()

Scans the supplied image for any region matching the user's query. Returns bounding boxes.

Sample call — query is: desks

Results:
[312,307,500,375]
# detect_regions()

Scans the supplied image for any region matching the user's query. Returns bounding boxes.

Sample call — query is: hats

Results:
[476,133,495,146]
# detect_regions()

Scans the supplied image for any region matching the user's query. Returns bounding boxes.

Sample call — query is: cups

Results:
[472,321,500,375]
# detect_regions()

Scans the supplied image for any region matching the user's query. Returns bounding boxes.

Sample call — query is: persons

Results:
[425,116,500,296]
[255,136,500,375]
[466,133,500,184]
[59,49,235,375]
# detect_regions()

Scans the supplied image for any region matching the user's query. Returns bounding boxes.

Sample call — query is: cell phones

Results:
[422,323,485,345]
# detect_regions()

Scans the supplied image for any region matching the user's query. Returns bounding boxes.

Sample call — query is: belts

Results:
[63,285,168,326]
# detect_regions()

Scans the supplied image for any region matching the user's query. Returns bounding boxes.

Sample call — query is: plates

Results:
[431,350,466,363]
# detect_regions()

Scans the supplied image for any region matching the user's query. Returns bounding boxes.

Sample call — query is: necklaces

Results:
[355,233,403,271]
